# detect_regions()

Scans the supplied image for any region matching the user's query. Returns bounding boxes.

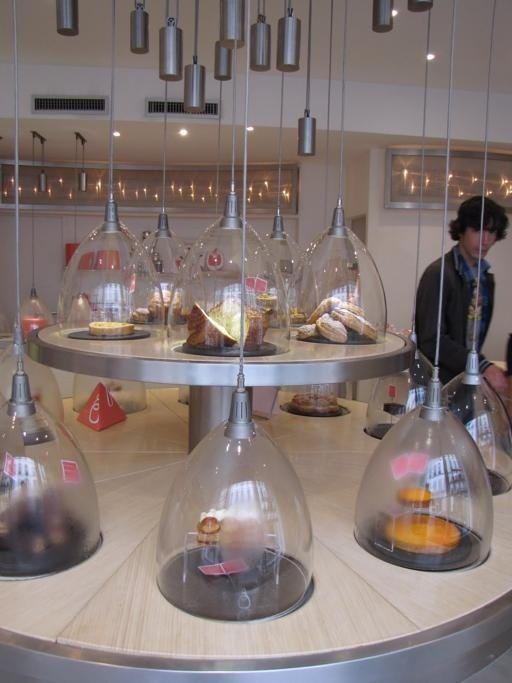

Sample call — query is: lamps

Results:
[276,2,300,72]
[214,41,233,82]
[185,3,206,110]
[129,1,150,55]
[249,4,270,71]
[371,1,393,32]
[298,4,317,157]
[159,2,182,82]
[407,1,435,14]
[220,0,244,48]
[55,0,80,37]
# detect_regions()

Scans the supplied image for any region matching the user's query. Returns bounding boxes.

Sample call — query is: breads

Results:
[88,321,135,337]
[385,511,461,557]
[184,290,278,352]
[132,290,181,326]
[197,507,262,549]
[395,487,434,510]
[290,297,377,345]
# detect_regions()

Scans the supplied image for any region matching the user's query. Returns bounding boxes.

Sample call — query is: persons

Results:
[416,196,511,424]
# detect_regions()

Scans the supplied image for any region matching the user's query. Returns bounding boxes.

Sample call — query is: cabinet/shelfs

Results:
[1,317,511,681]
